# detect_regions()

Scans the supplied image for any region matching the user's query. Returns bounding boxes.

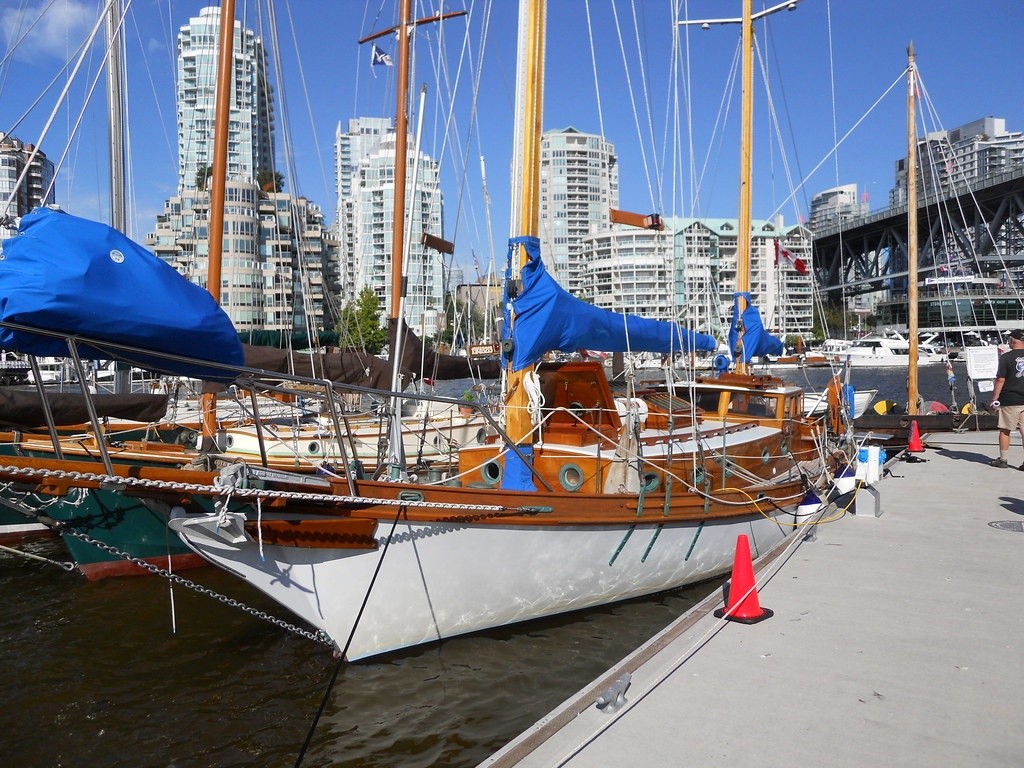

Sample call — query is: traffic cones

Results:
[905,420,926,452]
[713,535,774,624]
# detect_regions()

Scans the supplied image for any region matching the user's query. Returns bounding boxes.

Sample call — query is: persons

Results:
[989,329,1024,471]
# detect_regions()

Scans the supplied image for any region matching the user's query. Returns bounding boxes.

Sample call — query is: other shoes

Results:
[1019,462,1024,471]
[990,457,1008,468]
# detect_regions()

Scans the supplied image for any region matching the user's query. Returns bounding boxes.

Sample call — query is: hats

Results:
[1008,329,1024,342]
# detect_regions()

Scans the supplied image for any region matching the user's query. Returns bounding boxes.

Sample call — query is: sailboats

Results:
[0,3,1023,662]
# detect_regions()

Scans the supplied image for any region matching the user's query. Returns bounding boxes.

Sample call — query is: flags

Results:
[774,239,807,276]
[371,42,393,66]
